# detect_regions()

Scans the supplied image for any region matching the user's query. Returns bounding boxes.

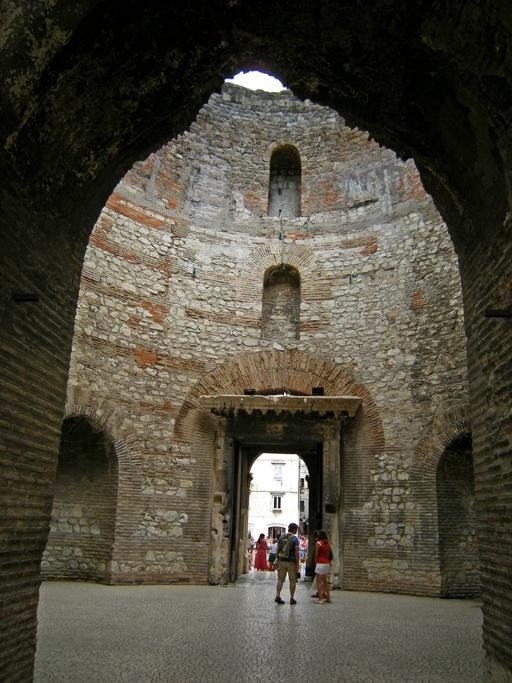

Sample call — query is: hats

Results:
[289,523,299,529]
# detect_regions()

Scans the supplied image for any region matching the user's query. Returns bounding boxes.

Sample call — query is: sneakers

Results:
[275,596,281,602]
[311,592,331,605]
[289,599,296,604]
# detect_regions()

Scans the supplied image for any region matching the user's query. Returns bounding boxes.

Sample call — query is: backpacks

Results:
[278,534,296,559]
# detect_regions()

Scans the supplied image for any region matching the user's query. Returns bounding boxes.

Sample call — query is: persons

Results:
[311,532,333,598]
[275,523,299,604]
[313,531,331,604]
[248,532,308,571]
